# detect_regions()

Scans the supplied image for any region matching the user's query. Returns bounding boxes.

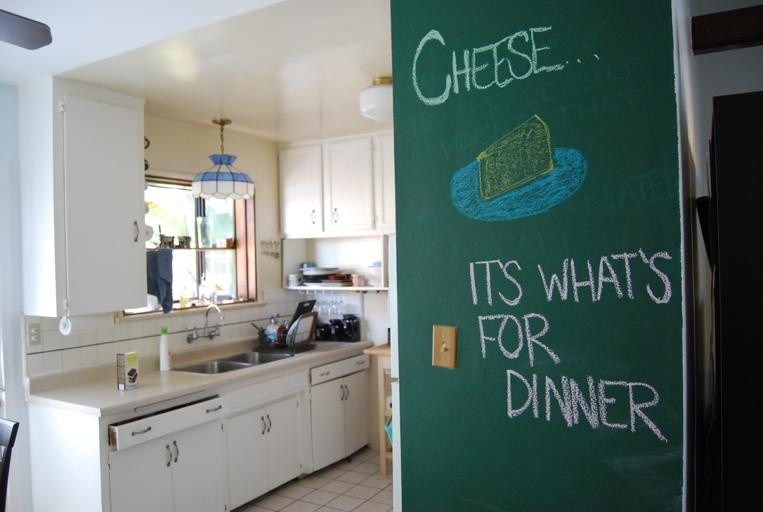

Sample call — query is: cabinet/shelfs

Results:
[308,351,375,475]
[17,74,148,319]
[26,394,226,512]
[225,368,307,512]
[278,133,379,240]
[381,129,395,235]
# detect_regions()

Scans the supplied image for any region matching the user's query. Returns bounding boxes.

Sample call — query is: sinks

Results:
[171,361,250,374]
[226,352,294,364]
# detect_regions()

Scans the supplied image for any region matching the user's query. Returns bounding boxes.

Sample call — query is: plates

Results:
[298,266,340,286]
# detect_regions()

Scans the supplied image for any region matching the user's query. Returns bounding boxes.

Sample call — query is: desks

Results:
[363,343,394,477]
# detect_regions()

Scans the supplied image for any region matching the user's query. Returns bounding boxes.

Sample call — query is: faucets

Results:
[205,305,224,325]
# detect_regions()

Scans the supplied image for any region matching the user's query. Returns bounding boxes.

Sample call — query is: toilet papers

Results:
[160,328,170,371]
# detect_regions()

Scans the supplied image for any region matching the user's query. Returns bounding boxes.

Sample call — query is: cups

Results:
[350,273,366,287]
[195,215,214,248]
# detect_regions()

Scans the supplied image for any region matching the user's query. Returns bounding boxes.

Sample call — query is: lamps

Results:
[191,118,255,200]
[359,76,393,122]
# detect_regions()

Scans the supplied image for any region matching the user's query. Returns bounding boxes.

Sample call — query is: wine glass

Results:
[296,290,344,316]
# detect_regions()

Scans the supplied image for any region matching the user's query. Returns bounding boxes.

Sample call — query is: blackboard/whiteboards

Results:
[390,0,687,512]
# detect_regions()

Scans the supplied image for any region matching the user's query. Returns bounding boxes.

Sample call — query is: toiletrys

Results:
[199,273,210,301]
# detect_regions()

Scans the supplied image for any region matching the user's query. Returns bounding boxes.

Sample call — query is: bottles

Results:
[158,326,172,373]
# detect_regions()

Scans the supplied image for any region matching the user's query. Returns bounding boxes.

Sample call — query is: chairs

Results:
[0,417,20,512]
